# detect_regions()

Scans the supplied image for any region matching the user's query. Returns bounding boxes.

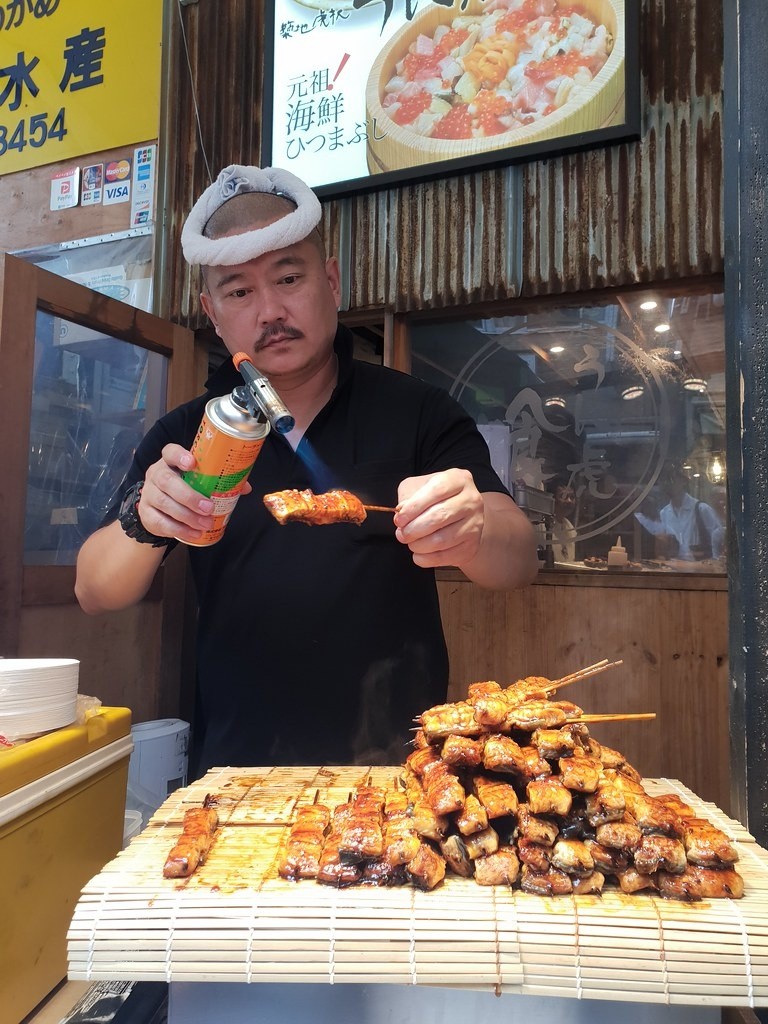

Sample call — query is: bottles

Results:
[608,535,628,565]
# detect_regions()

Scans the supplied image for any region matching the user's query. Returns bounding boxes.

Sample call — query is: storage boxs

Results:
[0,704,135,1024]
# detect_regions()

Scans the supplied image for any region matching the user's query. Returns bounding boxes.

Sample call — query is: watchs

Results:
[119,481,179,560]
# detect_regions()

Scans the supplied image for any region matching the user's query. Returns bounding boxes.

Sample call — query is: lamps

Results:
[621,385,645,400]
[705,438,728,485]
[544,396,567,409]
[683,377,709,393]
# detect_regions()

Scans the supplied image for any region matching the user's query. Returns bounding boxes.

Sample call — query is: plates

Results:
[0,654,81,735]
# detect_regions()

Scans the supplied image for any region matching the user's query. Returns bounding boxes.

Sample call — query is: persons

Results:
[660,474,722,561]
[537,478,577,563]
[635,498,695,562]
[69,192,540,787]
